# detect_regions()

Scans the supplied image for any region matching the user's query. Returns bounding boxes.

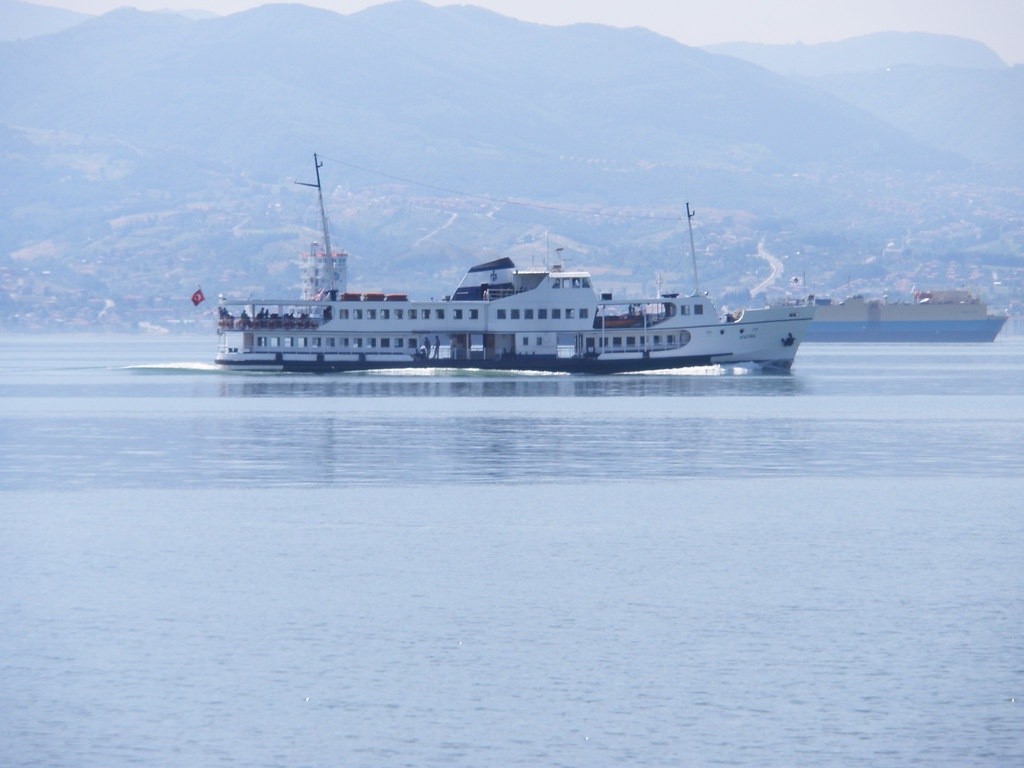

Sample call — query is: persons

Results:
[218,306,309,328]
[423,336,441,359]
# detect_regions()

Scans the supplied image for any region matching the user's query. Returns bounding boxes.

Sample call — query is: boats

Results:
[209,151,817,378]
[725,285,1011,344]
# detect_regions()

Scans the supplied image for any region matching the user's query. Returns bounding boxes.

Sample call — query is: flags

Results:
[191,289,204,306]
